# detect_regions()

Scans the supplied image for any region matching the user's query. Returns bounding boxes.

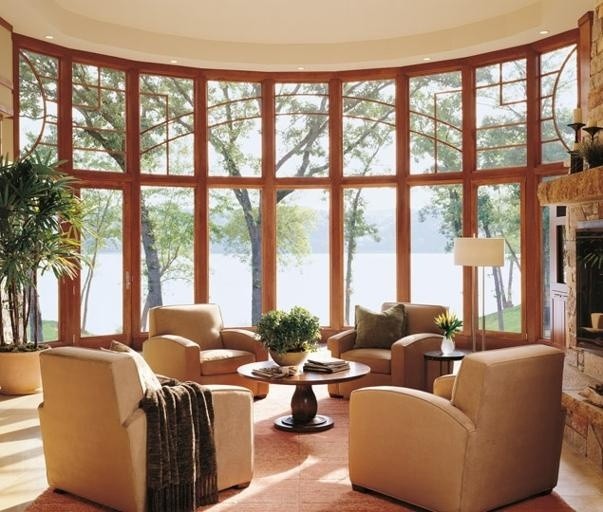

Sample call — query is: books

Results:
[303,357,351,374]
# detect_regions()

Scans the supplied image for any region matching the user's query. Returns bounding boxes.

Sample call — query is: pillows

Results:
[352,304,407,349]
[109,340,163,390]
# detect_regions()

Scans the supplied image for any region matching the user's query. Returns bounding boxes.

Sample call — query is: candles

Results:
[574,108,582,123]
[574,142,584,150]
[589,119,598,127]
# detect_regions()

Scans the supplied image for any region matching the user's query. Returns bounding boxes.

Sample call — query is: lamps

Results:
[453,237,505,350]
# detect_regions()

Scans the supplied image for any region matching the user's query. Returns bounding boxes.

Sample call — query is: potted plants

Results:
[253,307,322,365]
[0,145,98,396]
[434,310,464,354]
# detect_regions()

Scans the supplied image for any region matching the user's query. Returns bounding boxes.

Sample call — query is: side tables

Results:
[423,352,464,392]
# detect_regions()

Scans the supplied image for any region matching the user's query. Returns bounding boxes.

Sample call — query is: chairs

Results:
[143,304,269,398]
[38,345,253,512]
[327,303,453,399]
[348,344,567,512]
